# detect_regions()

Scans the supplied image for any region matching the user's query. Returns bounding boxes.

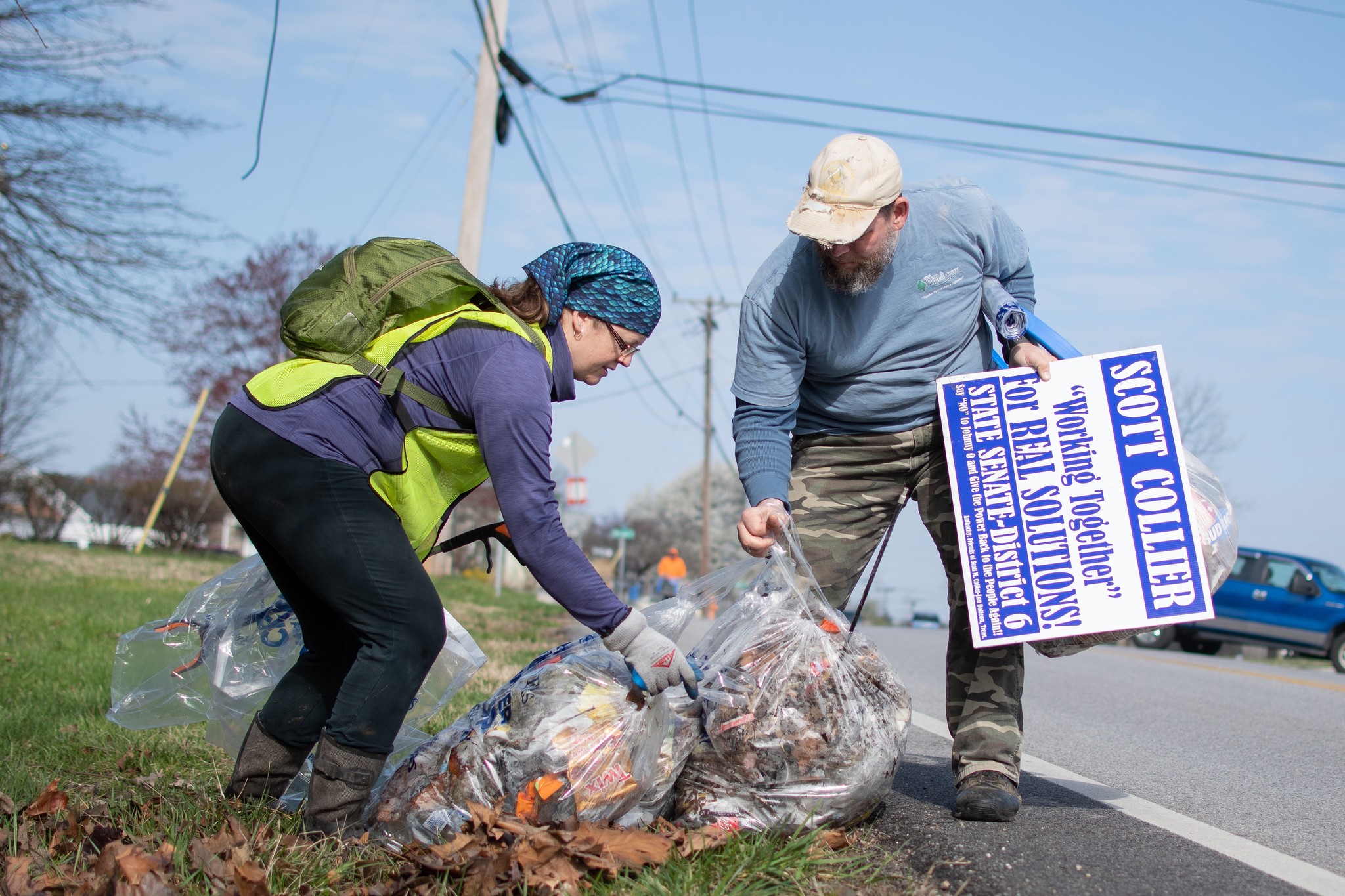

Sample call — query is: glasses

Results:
[604,320,641,358]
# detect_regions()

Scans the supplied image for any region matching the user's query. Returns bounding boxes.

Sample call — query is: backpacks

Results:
[278,235,548,428]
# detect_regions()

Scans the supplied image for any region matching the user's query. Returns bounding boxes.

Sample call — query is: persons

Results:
[658,547,687,598]
[209,242,698,850]
[729,131,1057,823]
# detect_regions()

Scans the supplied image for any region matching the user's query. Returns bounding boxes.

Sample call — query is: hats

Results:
[785,133,903,250]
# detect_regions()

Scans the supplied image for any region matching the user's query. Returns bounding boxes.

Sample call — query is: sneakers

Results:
[951,769,1023,823]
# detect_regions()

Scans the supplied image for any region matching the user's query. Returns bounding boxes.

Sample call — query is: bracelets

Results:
[1002,334,1040,364]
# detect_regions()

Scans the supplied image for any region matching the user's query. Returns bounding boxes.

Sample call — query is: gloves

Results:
[600,605,705,701]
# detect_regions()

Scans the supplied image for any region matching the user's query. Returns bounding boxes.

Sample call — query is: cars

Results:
[843,610,862,623]
[1131,546,1345,675]
[908,612,942,628]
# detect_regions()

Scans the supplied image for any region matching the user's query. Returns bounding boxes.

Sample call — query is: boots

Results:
[220,709,318,820]
[300,726,392,856]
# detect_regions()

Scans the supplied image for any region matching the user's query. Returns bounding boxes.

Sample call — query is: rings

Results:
[744,547,751,553]
